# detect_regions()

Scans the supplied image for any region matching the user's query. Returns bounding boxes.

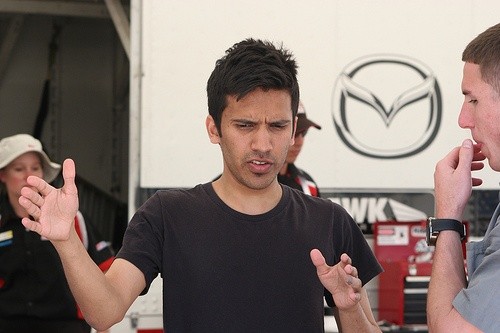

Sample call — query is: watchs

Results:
[425,214,467,247]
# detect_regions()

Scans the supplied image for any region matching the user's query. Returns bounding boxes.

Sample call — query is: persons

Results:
[276,99,321,197]
[0,133,117,333]
[18,38,385,333]
[425,22,500,333]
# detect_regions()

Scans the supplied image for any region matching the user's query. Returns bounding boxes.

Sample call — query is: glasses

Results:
[295,129,306,137]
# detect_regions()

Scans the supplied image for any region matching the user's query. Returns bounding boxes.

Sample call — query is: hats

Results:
[0,133,61,182]
[294,99,321,131]
